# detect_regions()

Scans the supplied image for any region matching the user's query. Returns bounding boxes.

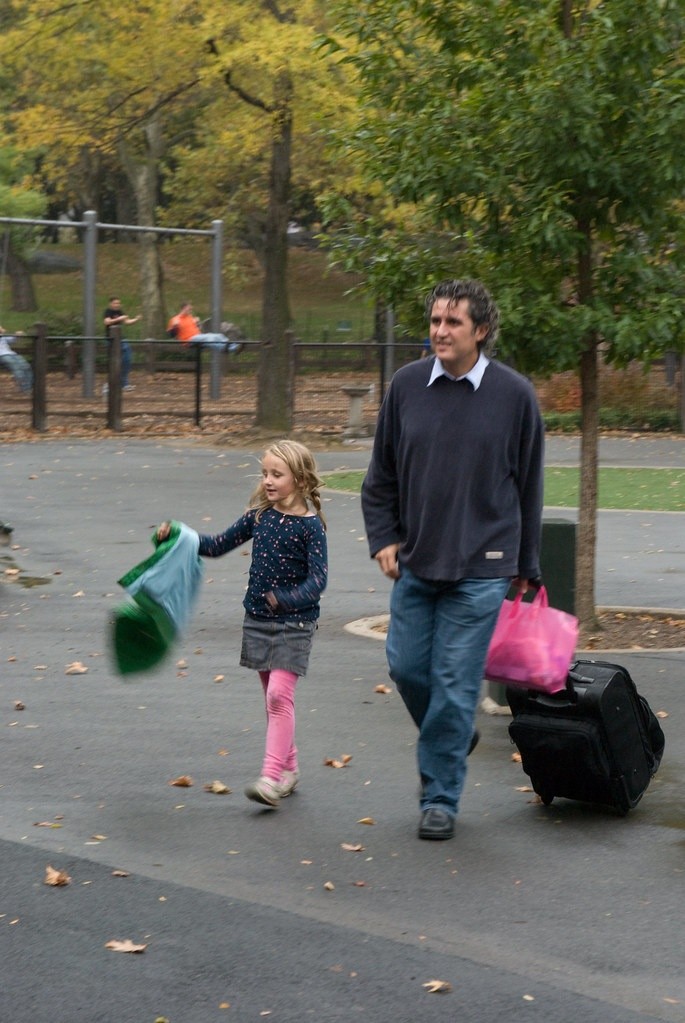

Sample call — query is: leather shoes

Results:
[466,731,479,758]
[418,807,453,839]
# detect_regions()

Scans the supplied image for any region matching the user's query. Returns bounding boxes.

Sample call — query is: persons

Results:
[102,295,143,391]
[155,441,328,809]
[167,301,242,354]
[361,277,545,839]
[0,326,34,395]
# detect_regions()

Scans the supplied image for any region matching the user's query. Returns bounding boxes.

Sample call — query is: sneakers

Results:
[243,777,281,807]
[279,768,300,797]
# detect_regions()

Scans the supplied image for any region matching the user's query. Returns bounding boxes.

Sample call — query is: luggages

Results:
[506,576,665,818]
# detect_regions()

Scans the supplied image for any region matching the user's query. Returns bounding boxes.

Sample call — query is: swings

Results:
[0,223,36,367]
[155,240,206,350]
[105,231,156,355]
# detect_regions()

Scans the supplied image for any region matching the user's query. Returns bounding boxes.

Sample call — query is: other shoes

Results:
[102,383,109,395]
[234,344,245,356]
[224,343,230,355]
[121,384,136,392]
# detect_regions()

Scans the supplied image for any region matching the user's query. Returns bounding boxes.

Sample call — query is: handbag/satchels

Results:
[484,584,579,695]
